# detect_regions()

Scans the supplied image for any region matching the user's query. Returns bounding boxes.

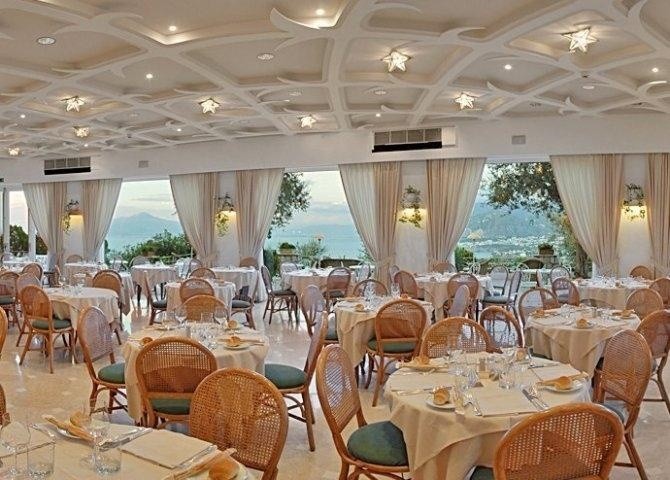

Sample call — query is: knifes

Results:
[522,389,543,411]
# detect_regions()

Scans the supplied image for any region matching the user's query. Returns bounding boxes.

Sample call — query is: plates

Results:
[225,343,249,349]
[620,315,637,319]
[426,398,455,410]
[548,382,583,392]
[572,322,594,328]
[58,419,101,439]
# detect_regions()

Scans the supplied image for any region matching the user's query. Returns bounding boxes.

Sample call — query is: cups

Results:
[27,433,56,480]
[94,433,120,473]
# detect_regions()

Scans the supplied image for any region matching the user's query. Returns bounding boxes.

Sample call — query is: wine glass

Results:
[80,400,110,468]
[0,414,30,480]
[58,275,84,296]
[162,305,227,352]
[364,282,400,309]
[446,334,530,392]
